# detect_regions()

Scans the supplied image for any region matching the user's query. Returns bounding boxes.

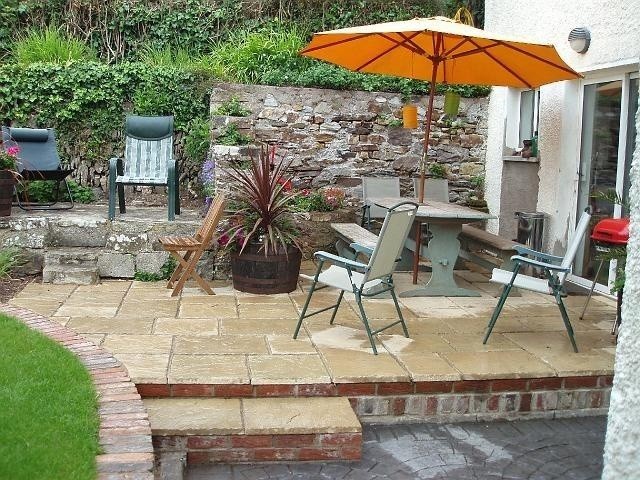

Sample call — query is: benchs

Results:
[419,219,530,301]
[329,220,402,284]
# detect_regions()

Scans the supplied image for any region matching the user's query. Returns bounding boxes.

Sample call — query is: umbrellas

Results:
[297,15,584,286]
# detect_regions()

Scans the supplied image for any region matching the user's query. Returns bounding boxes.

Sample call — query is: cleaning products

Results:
[530,130,538,157]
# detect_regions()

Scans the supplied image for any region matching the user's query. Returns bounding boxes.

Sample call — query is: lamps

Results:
[568,28,592,55]
[400,48,419,129]
[443,57,460,116]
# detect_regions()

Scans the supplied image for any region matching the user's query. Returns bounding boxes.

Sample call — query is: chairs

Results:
[477,204,591,356]
[158,193,225,298]
[2,123,80,213]
[106,111,182,220]
[360,176,402,228]
[412,176,452,204]
[290,201,420,355]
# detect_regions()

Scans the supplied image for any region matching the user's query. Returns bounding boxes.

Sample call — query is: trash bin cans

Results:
[589,217,631,287]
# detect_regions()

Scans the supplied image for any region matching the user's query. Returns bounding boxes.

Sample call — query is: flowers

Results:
[212,145,314,261]
[0,139,22,177]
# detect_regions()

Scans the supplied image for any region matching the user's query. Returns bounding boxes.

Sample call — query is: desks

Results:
[371,194,500,298]
